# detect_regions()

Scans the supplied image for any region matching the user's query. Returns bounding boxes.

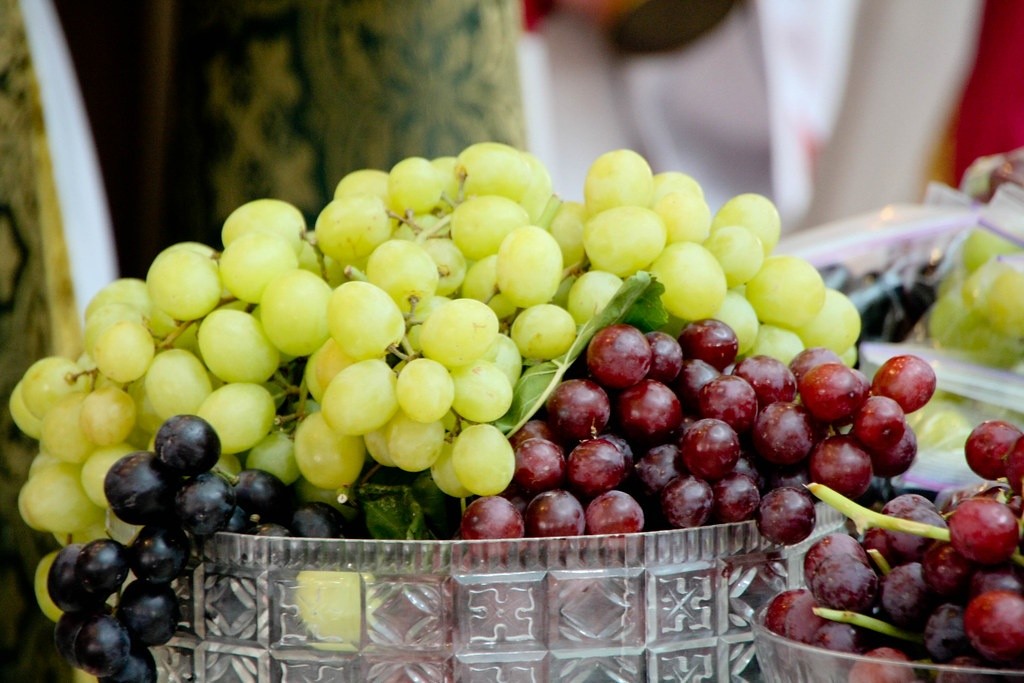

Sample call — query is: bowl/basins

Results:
[151,501,851,683]
[751,587,1024,683]
[860,340,1023,494]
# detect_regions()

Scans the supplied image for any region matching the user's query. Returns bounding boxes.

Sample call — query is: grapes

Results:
[8,143,1024,683]
